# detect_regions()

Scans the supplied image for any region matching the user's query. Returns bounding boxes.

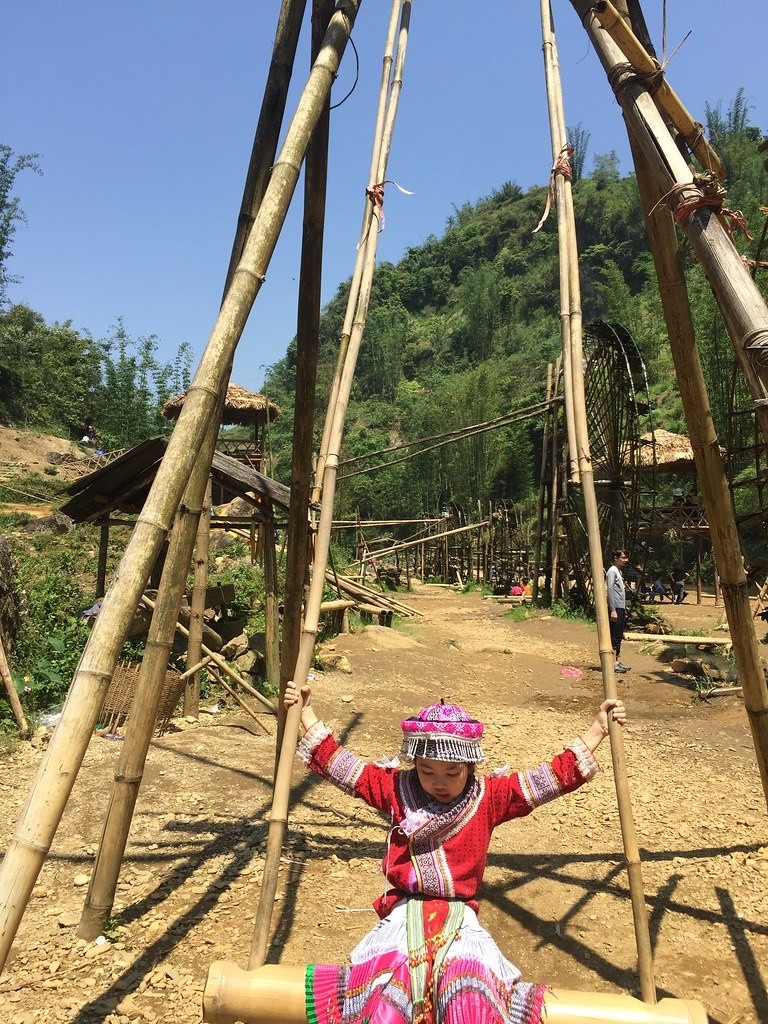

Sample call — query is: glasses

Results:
[620,557,628,559]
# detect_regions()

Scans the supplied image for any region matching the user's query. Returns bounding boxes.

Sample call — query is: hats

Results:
[398,697,486,763]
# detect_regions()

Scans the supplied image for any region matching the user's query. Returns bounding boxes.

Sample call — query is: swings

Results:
[199,0,709,1024]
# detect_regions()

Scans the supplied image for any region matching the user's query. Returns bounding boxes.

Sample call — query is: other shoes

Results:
[615,662,631,673]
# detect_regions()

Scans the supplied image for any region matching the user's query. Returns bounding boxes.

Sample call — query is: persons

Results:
[670,572,690,604]
[653,574,667,594]
[505,578,530,600]
[606,548,631,673]
[624,581,637,610]
[283,681,626,1023]
[636,575,657,605]
